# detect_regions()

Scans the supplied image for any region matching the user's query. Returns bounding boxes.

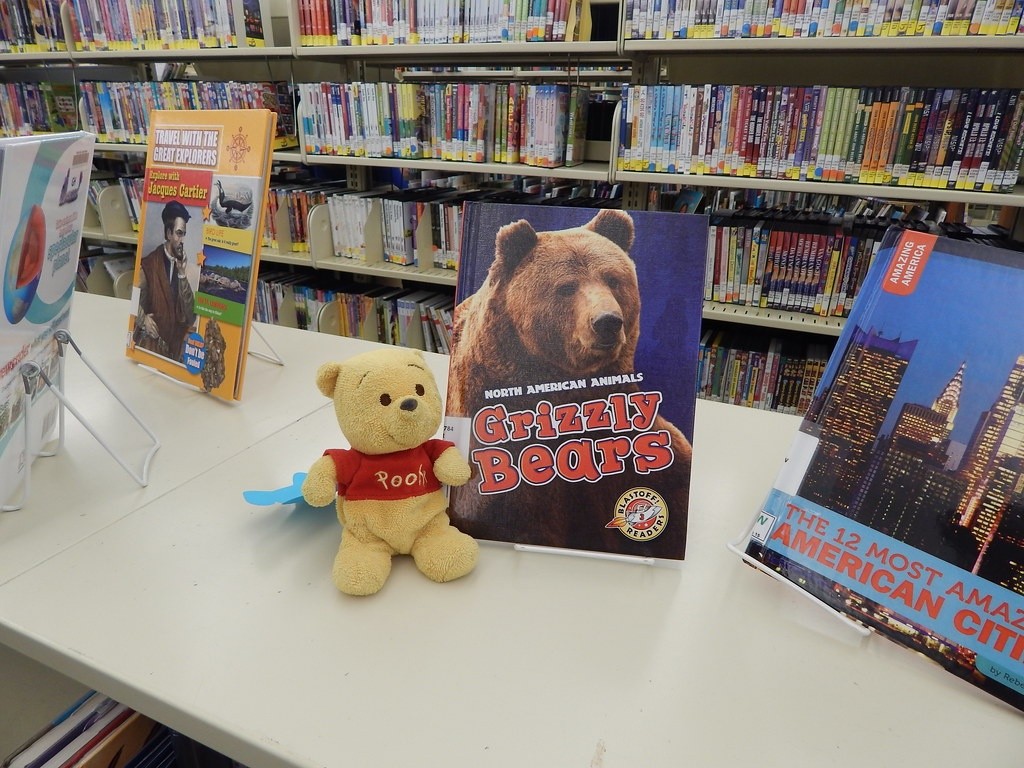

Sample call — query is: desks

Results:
[0,292,1024,768]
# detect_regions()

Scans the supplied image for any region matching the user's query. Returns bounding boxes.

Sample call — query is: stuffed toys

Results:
[302,349,478,596]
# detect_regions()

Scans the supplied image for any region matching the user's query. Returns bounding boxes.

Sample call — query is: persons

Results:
[133,201,196,363]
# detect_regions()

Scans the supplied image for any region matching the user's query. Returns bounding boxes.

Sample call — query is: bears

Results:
[445,208,692,545]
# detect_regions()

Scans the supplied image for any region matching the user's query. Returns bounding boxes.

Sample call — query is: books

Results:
[0,0,1023,768]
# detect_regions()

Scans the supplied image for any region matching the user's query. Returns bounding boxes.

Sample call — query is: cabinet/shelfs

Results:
[0,35,1024,415]
